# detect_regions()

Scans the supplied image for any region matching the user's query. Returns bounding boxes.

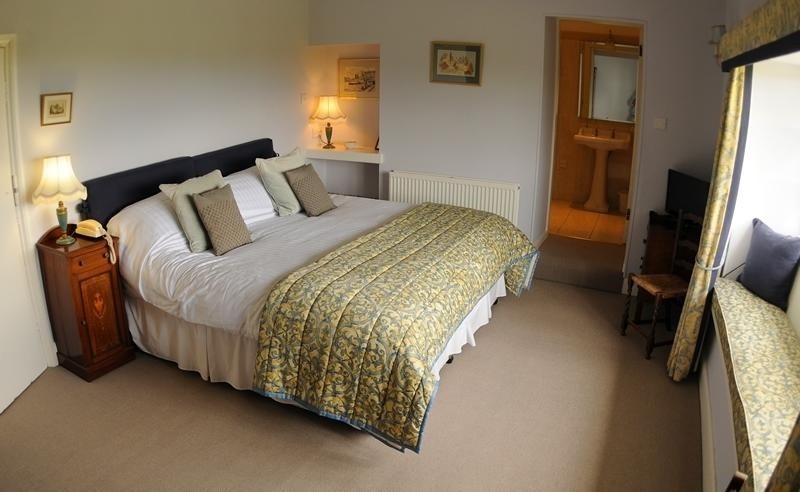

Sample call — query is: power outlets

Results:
[312,128,322,138]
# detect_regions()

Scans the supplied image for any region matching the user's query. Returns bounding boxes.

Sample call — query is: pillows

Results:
[736,216,800,314]
[284,163,337,218]
[254,146,309,216]
[193,182,251,257]
[159,169,226,254]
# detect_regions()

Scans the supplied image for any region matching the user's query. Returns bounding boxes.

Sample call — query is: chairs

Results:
[618,208,704,360]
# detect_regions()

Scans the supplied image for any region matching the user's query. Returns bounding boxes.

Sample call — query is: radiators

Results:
[386,169,520,230]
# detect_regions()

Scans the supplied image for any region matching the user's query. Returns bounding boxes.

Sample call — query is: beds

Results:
[79,137,539,457]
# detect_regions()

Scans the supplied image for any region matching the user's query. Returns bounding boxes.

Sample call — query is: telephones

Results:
[76,219,105,238]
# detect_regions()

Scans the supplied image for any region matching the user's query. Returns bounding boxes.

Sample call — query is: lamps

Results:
[310,95,346,149]
[29,151,90,245]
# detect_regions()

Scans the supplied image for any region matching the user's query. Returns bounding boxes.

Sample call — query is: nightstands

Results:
[34,223,137,383]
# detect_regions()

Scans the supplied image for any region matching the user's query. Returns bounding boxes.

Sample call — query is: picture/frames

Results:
[40,91,73,126]
[429,40,485,87]
[338,57,380,102]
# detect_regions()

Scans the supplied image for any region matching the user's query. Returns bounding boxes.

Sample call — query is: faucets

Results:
[594,126,598,136]
[611,128,616,139]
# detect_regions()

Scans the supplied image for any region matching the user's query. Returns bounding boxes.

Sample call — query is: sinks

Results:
[574,127,632,151]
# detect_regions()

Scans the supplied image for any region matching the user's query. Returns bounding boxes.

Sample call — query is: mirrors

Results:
[578,41,640,125]
